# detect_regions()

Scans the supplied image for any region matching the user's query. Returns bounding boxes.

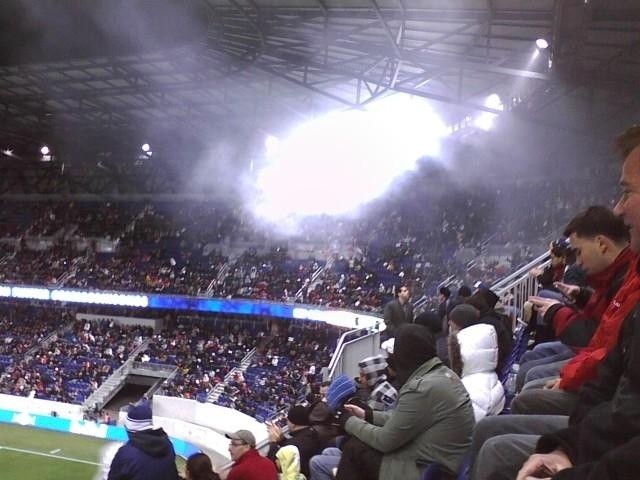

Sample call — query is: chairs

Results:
[456,325,530,414]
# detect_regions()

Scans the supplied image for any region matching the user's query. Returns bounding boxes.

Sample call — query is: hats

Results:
[225,431,255,446]
[448,303,481,328]
[414,311,441,332]
[124,406,152,433]
[287,405,307,426]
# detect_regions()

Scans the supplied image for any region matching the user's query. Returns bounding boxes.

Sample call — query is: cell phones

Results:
[554,284,573,301]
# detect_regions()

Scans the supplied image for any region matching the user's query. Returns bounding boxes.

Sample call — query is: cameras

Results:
[552,238,568,251]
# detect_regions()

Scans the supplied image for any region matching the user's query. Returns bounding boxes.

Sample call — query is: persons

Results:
[1,123,639,479]
